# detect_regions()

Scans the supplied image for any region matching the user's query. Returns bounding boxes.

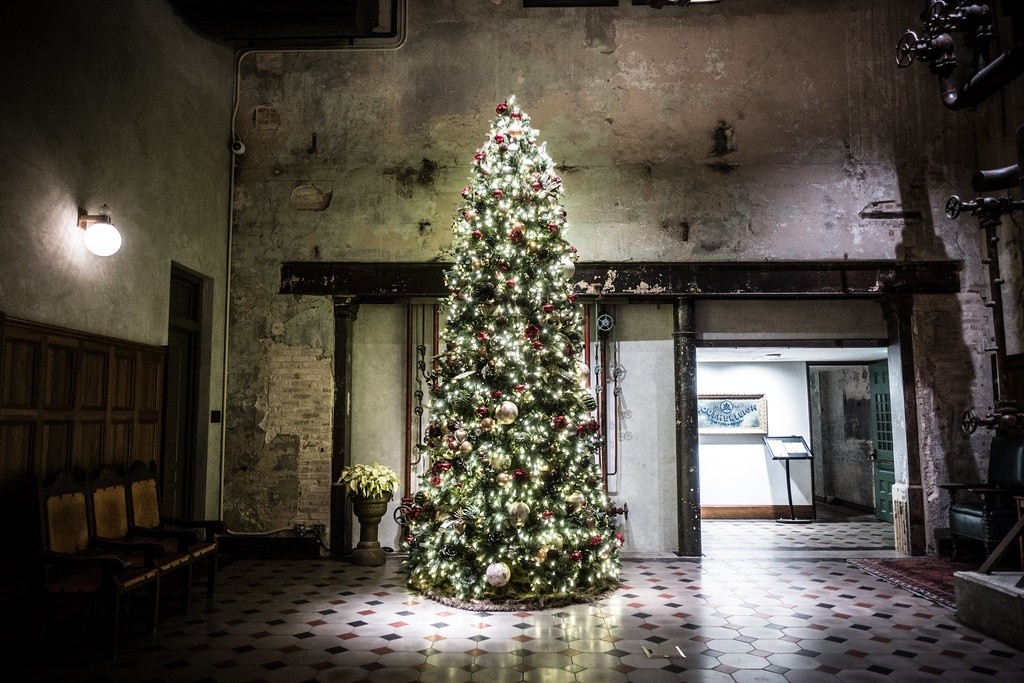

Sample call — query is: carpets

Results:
[845,552,976,612]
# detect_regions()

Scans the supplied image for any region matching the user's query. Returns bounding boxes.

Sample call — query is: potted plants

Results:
[333,462,394,567]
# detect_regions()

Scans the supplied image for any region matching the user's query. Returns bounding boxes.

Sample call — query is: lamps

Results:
[77,207,121,256]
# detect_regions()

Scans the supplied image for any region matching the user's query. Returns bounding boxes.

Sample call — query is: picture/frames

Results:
[696,394,768,434]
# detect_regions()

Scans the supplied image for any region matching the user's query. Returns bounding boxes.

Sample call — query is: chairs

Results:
[37,463,228,656]
